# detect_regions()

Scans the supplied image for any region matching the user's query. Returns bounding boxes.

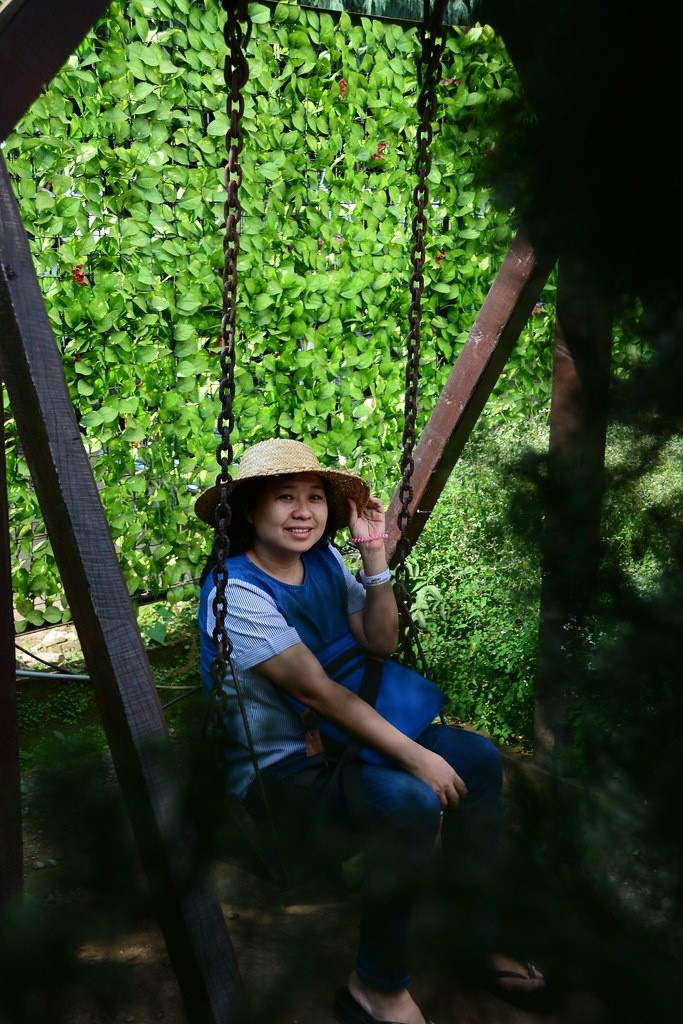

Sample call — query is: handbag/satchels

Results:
[278,632,447,766]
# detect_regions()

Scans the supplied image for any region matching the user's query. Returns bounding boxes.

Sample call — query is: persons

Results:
[197,439,545,1023]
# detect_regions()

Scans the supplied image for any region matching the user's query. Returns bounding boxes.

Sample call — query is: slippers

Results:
[450,940,558,1013]
[333,986,431,1024]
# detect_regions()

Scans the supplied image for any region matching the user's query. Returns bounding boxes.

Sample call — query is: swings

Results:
[198,0,451,882]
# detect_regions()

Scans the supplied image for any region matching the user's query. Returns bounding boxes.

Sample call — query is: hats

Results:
[195,439,371,545]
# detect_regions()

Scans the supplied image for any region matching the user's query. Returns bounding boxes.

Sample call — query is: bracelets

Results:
[359,567,392,585]
[347,534,390,543]
